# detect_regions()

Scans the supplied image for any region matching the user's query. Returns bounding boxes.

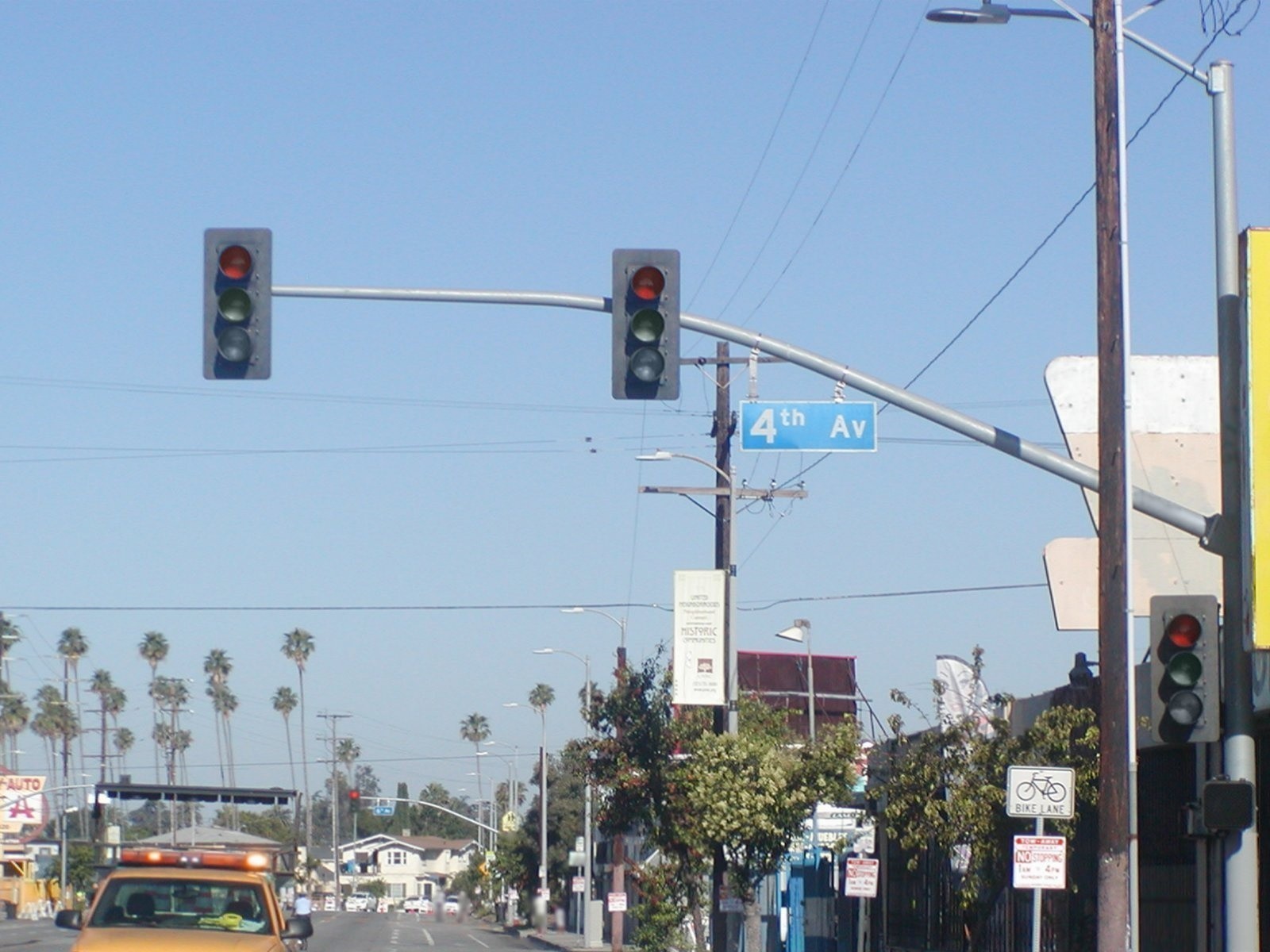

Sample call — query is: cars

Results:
[53,845,315,952]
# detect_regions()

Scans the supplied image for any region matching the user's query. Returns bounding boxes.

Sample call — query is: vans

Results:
[344,891,461,916]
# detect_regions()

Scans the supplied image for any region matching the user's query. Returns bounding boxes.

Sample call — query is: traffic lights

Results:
[204,227,273,382]
[611,247,680,401]
[349,789,361,814]
[1202,780,1255,832]
[1149,593,1221,743]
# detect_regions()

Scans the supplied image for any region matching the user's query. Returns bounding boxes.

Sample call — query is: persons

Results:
[290,892,311,945]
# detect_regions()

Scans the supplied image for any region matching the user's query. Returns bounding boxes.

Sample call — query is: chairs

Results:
[118,893,161,924]
[227,901,253,921]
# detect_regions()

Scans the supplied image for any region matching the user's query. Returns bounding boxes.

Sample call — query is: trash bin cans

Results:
[495,901,506,922]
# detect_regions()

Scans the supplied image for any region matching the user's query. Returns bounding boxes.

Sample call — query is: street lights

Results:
[561,607,627,745]
[775,618,817,759]
[457,740,519,910]
[501,701,550,934]
[635,451,739,741]
[925,4,1261,952]
[533,647,594,937]
